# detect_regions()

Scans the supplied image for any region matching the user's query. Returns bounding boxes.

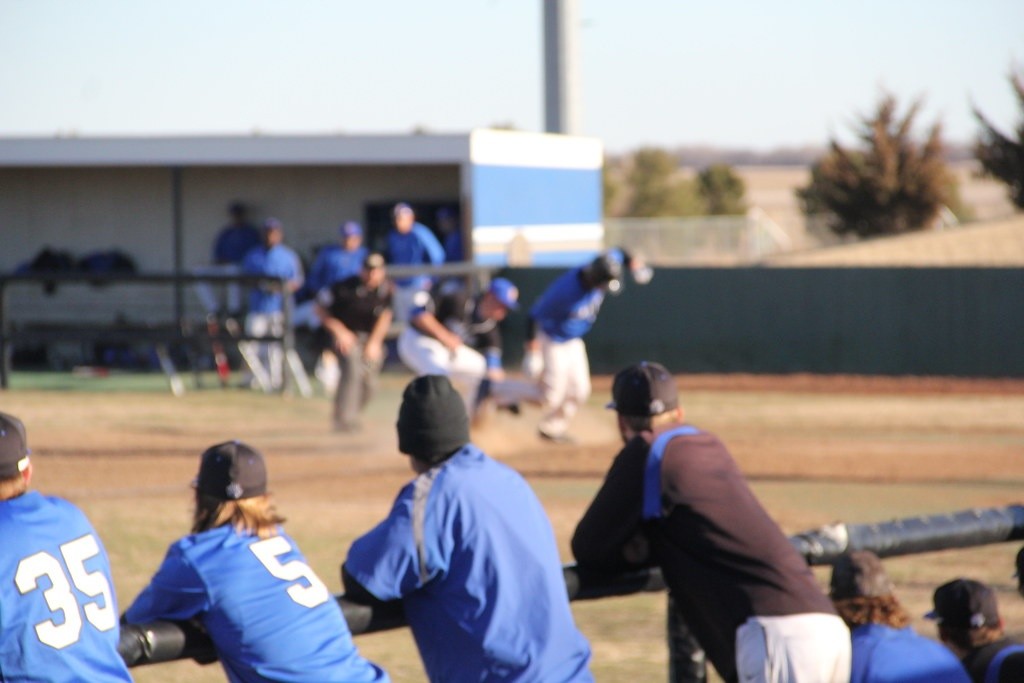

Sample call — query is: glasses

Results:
[1,412,29,477]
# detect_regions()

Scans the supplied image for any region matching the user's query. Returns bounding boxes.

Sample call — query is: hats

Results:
[258,220,281,233]
[190,440,268,503]
[606,361,678,417]
[339,223,362,238]
[831,551,887,599]
[925,580,998,629]
[397,375,470,457]
[593,256,627,298]
[485,279,521,315]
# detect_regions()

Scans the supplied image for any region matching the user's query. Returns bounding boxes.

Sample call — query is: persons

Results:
[340,374,593,683]
[312,253,399,422]
[0,412,135,683]
[830,550,971,682]
[208,200,446,394]
[120,441,388,683]
[396,277,520,426]
[520,246,650,440]
[922,579,1024,683]
[571,361,853,683]
